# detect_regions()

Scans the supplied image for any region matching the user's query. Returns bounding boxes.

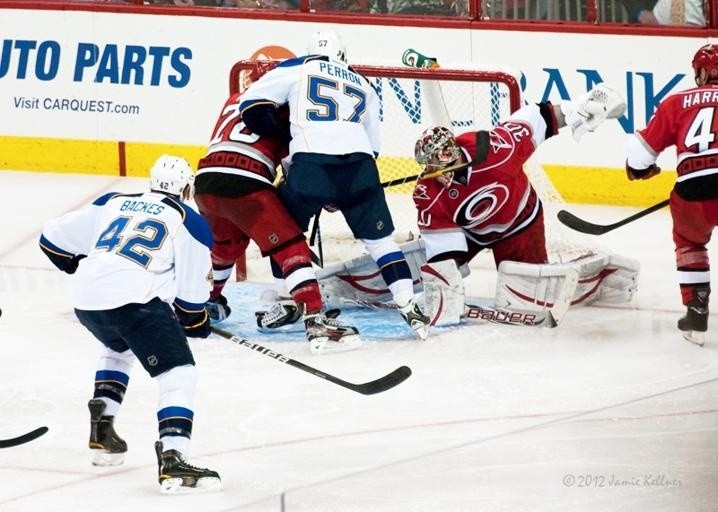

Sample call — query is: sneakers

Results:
[677,291,710,332]
[155,440,218,486]
[88,399,127,453]
[398,296,431,332]
[260,305,298,330]
[206,305,231,323]
[208,295,226,307]
[304,312,359,342]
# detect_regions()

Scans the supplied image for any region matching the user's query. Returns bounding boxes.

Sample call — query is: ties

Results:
[670,0,685,24]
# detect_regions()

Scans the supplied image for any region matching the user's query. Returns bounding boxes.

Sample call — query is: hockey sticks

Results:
[557,195,669,237]
[201,318,411,396]
[0,426,49,450]
[381,131,489,189]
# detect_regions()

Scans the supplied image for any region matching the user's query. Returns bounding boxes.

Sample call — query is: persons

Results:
[191,60,358,340]
[238,30,430,331]
[626,45,717,331]
[411,81,641,320]
[39,153,221,489]
[372,0,718,25]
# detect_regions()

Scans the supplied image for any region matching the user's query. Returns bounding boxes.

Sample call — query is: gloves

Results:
[627,159,660,181]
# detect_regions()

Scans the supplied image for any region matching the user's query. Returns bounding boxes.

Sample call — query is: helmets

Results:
[150,155,196,202]
[250,61,281,83]
[306,31,348,64]
[415,126,462,190]
[690,44,718,83]
